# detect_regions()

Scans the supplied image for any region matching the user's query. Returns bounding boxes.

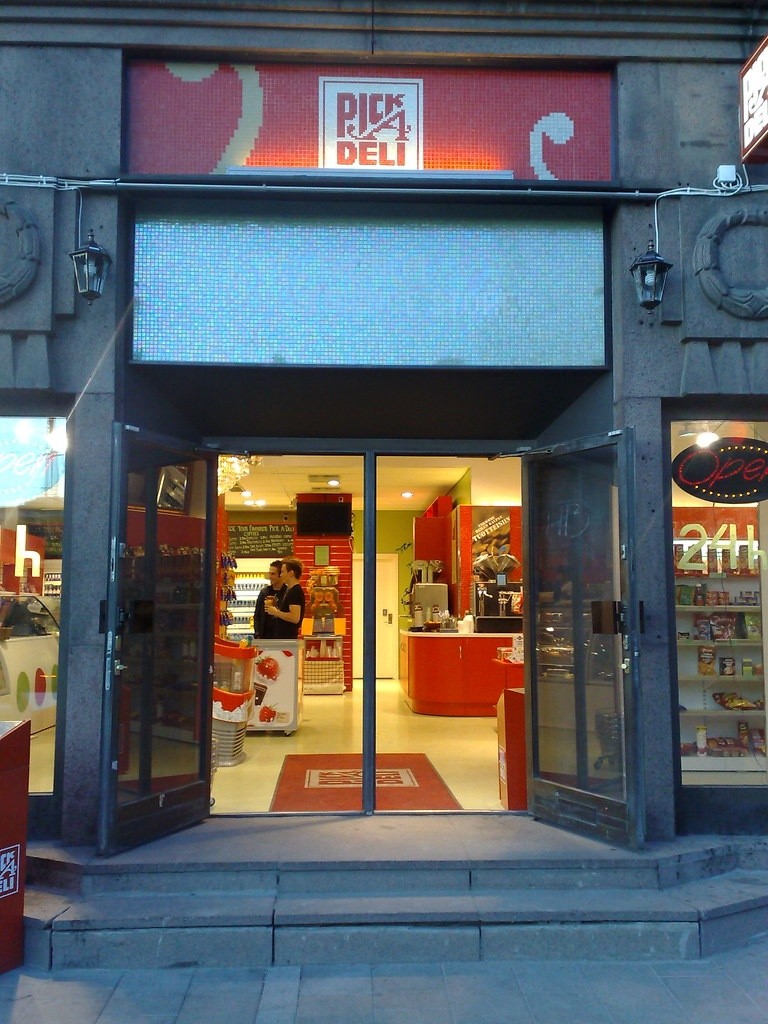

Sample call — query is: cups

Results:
[265,596,276,612]
[421,566,434,583]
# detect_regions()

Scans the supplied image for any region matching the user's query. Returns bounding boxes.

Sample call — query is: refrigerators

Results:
[245,640,303,736]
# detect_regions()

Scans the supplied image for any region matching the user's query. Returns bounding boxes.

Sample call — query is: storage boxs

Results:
[207,635,258,764]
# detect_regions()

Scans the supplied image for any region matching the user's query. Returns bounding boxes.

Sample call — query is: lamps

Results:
[68,229,112,306]
[629,239,674,308]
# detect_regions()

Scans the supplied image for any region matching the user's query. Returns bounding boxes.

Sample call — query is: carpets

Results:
[268,752,463,811]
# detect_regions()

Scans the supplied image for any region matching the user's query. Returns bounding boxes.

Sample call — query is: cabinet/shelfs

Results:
[398,632,409,695]
[674,572,768,785]
[227,572,271,628]
[43,571,62,600]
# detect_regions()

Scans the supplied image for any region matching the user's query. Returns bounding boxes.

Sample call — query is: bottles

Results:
[45,575,61,597]
[695,584,705,606]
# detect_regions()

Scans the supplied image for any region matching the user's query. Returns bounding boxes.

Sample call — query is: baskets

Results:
[212,716,247,766]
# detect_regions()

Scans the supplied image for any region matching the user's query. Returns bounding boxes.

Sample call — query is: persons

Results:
[254,556,305,640]
